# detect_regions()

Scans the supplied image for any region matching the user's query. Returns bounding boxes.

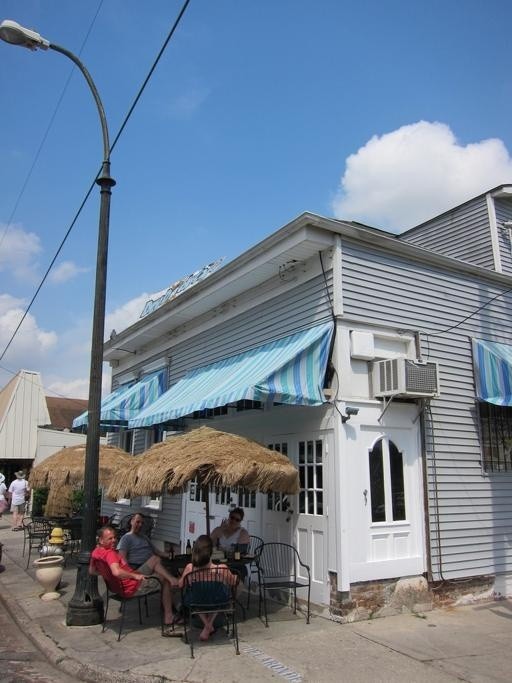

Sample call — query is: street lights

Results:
[0,19,117,629]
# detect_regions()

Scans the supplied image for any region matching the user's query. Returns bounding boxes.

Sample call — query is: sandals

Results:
[12,526,22,531]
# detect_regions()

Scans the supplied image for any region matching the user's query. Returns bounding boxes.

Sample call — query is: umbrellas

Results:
[44,486,73,519]
[104,424,303,538]
[28,442,133,488]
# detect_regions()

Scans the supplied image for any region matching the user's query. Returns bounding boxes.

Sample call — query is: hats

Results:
[15,471,26,479]
[0,473,5,482]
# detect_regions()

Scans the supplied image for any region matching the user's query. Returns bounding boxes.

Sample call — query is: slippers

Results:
[164,617,185,626]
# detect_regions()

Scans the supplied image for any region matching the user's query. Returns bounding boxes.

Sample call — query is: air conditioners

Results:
[371,356,440,400]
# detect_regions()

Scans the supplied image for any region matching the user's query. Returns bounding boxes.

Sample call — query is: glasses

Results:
[229,516,241,523]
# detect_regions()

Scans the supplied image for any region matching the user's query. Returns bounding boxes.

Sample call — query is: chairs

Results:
[254,542,311,627]
[175,566,240,658]
[89,556,164,642]
[242,535,264,610]
[21,513,155,570]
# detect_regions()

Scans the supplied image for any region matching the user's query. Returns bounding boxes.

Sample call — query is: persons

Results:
[8,470,31,531]
[115,512,178,577]
[178,535,234,641]
[0,472,9,521]
[209,507,249,602]
[88,526,186,625]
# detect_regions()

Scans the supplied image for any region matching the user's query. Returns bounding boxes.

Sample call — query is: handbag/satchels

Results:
[25,494,30,501]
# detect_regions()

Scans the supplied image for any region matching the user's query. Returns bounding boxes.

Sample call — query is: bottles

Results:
[186,538,192,555]
[169,545,175,561]
[234,542,241,561]
[216,537,221,548]
